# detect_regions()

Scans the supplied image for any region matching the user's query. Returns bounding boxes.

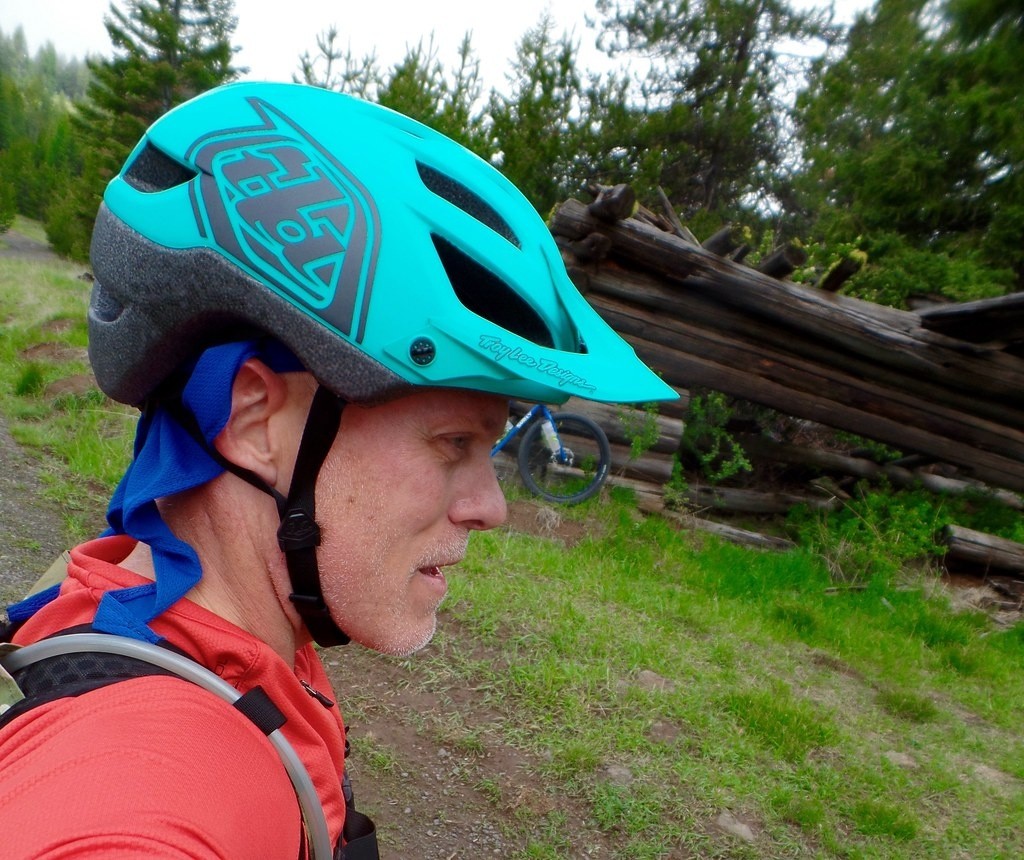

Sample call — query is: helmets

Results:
[86,80,683,403]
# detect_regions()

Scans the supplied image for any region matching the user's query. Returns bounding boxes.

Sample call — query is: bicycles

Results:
[488,404,611,507]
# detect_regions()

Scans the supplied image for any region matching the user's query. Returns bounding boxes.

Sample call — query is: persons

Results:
[0,80,680,860]
